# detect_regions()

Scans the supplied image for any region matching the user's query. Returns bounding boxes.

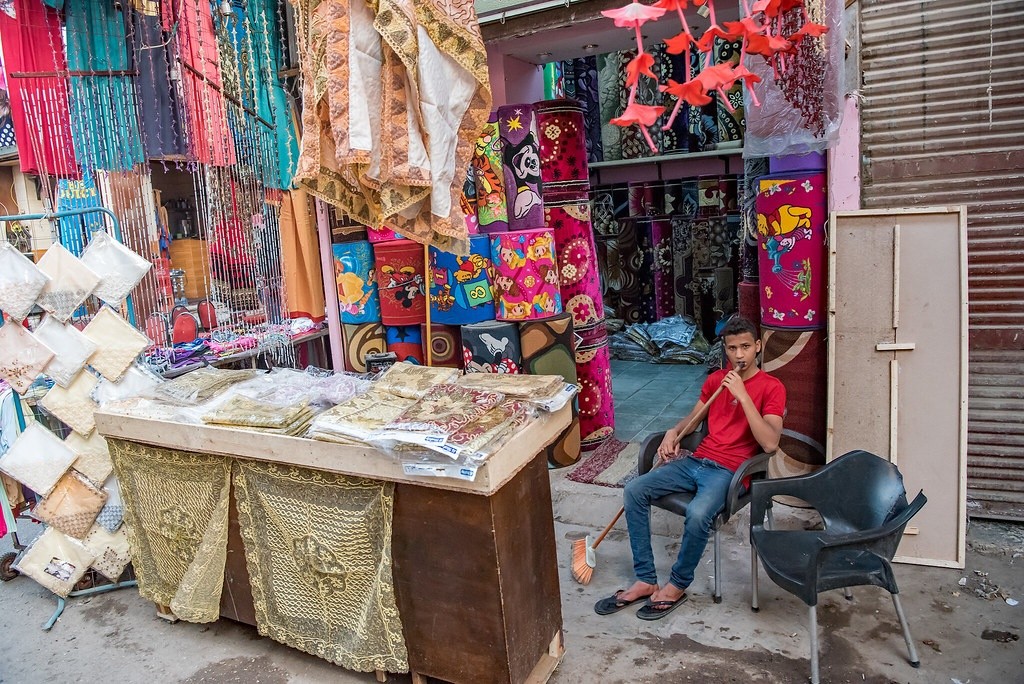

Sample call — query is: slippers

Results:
[593,590,648,615]
[636,593,688,620]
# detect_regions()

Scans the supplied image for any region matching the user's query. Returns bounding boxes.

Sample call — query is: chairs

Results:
[638,408,777,605]
[748,449,928,684]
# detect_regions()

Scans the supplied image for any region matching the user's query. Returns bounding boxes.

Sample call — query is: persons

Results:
[594,320,785,619]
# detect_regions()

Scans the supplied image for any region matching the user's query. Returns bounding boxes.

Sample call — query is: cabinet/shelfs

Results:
[96,372,572,684]
